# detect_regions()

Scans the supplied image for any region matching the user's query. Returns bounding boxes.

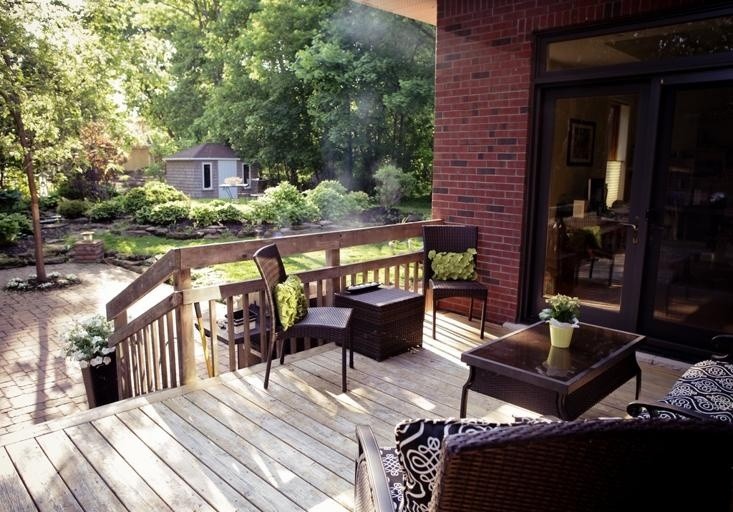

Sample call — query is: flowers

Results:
[64,313,116,363]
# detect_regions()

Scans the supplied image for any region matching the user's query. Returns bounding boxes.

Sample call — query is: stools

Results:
[335,282,424,361]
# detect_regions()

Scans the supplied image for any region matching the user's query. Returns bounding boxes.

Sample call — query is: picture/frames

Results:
[566,118,597,168]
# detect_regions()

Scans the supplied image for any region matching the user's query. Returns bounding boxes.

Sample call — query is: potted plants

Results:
[540,293,580,348]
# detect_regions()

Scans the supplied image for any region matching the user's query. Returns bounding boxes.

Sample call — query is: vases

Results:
[80,350,119,409]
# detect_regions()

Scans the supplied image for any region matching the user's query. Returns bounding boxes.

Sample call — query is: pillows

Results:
[585,225,602,247]
[428,248,478,281]
[275,274,307,330]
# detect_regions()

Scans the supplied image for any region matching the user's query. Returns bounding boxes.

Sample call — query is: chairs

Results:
[564,226,615,286]
[355,422,733,512]
[252,244,354,392]
[422,224,488,339]
[627,330,733,423]
